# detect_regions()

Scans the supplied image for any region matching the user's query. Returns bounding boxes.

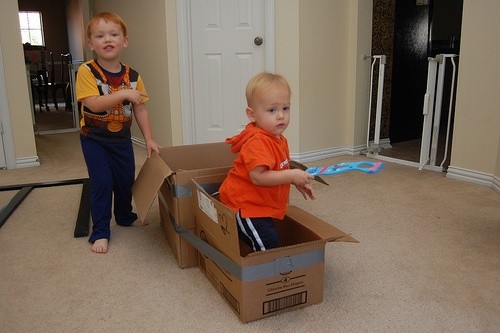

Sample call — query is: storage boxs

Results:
[130,139,329,270]
[190,172,361,324]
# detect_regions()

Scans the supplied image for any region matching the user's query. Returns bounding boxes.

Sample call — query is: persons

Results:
[76,10,162,254]
[220,71,318,250]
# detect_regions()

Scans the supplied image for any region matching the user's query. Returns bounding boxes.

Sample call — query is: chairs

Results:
[47,53,73,113]
[23,41,58,113]
